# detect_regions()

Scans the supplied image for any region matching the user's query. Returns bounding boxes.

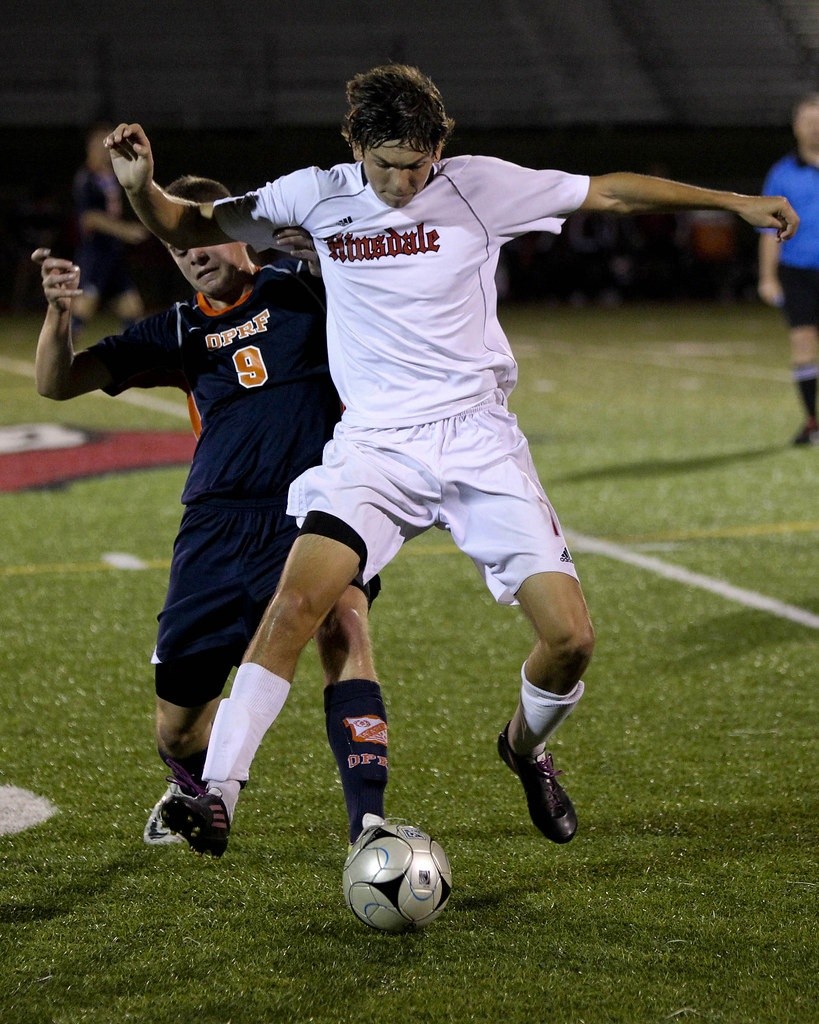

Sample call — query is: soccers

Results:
[340,823,456,934]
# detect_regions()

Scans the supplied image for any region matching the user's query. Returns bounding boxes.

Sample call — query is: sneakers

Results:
[165,758,230,860]
[497,720,578,844]
[144,778,203,845]
[348,813,407,855]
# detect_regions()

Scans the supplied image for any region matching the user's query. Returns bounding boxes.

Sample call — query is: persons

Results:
[752,92,819,442]
[102,63,801,856]
[63,125,151,336]
[30,172,397,871]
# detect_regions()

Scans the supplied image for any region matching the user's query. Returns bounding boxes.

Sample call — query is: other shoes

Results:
[795,426,819,444]
